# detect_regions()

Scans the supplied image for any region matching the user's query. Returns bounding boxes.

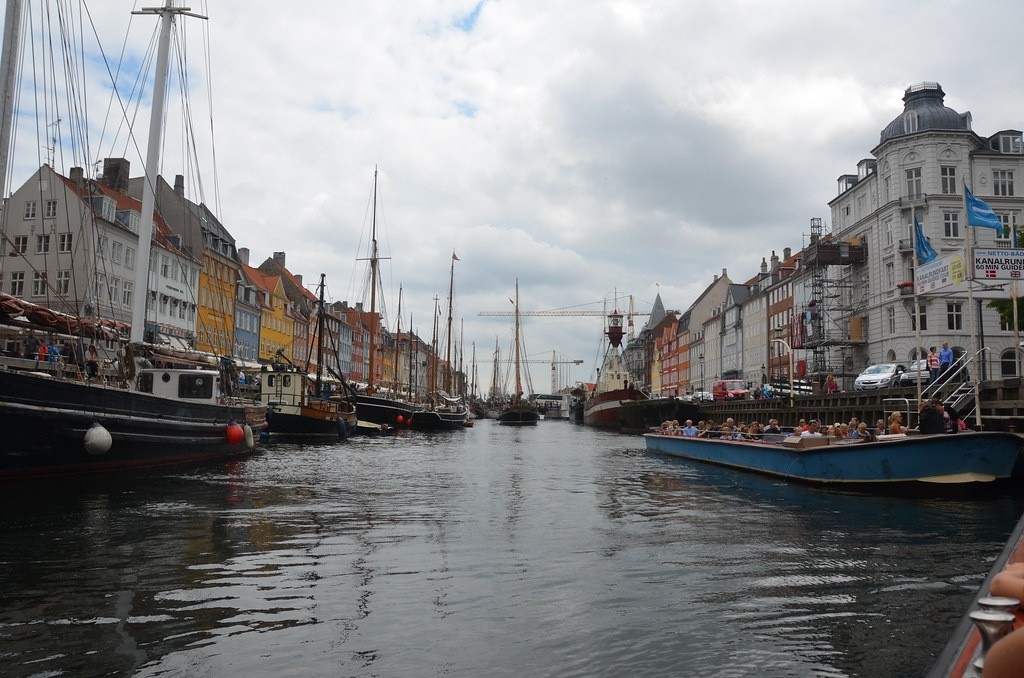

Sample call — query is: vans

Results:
[713,378,748,402]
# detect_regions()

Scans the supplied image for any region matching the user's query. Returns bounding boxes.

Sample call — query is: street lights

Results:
[774,324,784,397]
[760,363,767,386]
[699,353,706,402]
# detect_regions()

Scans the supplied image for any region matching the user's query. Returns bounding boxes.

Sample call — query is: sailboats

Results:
[0,1,538,469]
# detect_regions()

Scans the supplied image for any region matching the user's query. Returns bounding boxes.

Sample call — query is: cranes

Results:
[475,293,681,341]
[469,349,584,394]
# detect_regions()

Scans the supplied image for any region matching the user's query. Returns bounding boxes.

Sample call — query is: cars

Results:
[900,358,970,386]
[853,364,906,390]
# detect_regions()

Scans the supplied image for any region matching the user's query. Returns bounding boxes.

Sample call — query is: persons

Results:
[982,626,1024,678]
[988,562,1024,607]
[21,328,86,367]
[656,398,974,444]
[937,341,954,384]
[84,345,98,380]
[927,346,940,386]
[825,374,841,397]
[752,383,777,401]
[237,368,245,384]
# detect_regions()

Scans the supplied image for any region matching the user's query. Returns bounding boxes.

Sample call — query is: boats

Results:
[583,288,641,428]
[643,422,1023,498]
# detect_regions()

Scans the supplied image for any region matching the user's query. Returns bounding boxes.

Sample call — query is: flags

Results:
[913,215,939,263]
[963,183,1004,237]
